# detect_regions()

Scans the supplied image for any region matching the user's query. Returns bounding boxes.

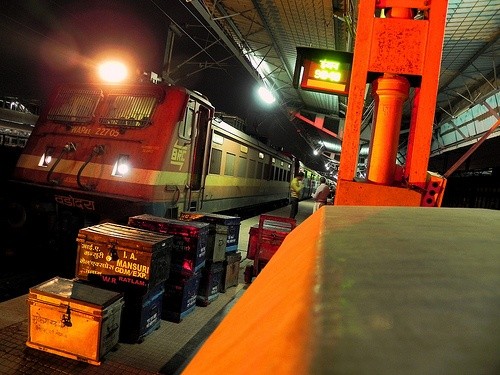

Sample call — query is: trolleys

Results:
[244,215,298,284]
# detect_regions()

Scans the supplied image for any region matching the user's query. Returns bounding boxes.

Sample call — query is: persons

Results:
[311,177,330,213]
[289,172,305,219]
[329,182,336,199]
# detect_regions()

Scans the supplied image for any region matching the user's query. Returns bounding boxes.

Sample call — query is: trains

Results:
[9,65,323,228]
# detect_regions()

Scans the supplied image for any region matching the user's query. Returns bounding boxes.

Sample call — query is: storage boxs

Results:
[27,211,242,366]
